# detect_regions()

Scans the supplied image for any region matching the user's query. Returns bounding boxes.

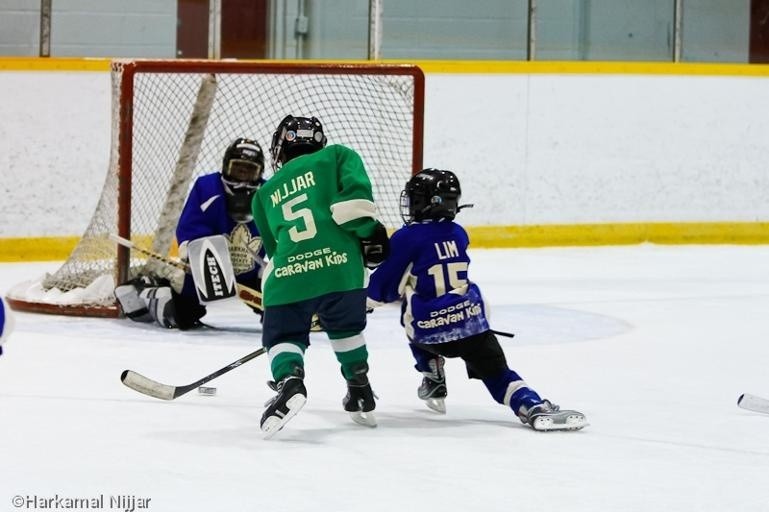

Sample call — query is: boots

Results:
[418,358,447,399]
[509,386,587,432]
[340,362,376,412]
[260,372,307,433]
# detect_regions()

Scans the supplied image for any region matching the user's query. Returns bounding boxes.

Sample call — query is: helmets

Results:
[223,138,264,180]
[406,167,461,199]
[269,115,328,151]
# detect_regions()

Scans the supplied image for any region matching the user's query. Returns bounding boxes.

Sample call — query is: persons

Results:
[249,113,390,435]
[114,136,327,336]
[364,165,594,435]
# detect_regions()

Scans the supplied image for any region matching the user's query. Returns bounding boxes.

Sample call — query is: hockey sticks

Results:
[109,233,318,323]
[120,321,318,400]
